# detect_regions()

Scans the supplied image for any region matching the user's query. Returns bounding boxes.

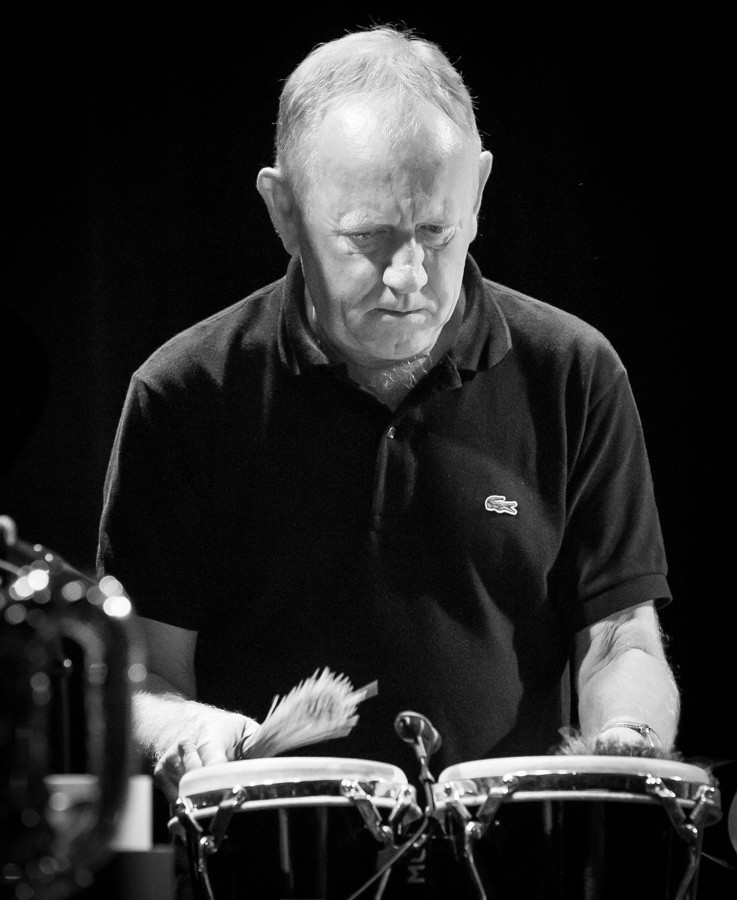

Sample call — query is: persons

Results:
[98,29,680,793]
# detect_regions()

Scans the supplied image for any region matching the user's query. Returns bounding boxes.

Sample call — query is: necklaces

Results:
[312,304,430,371]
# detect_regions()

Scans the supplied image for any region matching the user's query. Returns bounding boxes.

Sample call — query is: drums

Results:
[167,757,423,900]
[427,755,722,900]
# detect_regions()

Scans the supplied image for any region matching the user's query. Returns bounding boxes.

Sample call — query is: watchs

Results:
[599,721,664,749]
